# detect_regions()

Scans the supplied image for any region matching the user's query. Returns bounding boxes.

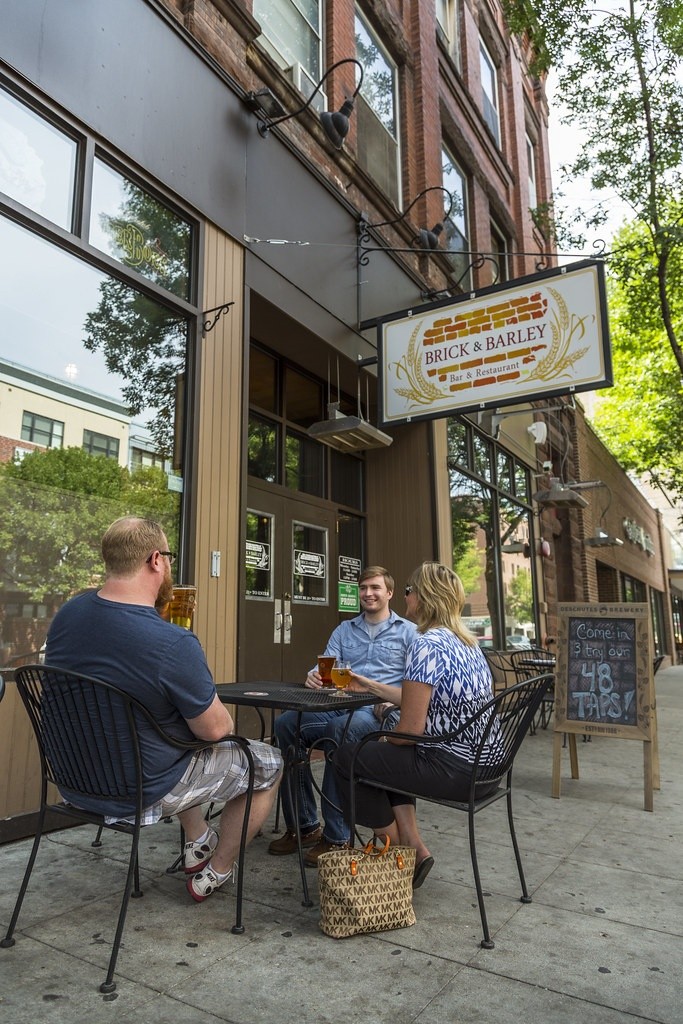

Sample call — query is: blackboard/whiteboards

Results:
[552,602,658,742]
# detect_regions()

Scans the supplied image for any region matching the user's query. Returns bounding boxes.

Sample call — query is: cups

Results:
[328,661,352,699]
[316,654,338,689]
[170,585,198,632]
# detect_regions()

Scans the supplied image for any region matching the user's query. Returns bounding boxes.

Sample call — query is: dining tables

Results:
[214,680,389,908]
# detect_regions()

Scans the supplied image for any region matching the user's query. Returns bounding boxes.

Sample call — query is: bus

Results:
[0,584,97,676]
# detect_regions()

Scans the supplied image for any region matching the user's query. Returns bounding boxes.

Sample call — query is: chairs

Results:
[481,646,544,727]
[346,673,556,949]
[655,655,665,674]
[0,664,256,993]
[380,704,401,730]
[510,650,556,736]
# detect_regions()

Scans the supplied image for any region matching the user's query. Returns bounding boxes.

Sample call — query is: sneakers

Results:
[267,825,321,857]
[303,836,350,870]
[182,823,224,874]
[185,860,238,903]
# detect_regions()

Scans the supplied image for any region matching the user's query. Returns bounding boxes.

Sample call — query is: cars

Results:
[504,635,536,651]
[475,635,520,654]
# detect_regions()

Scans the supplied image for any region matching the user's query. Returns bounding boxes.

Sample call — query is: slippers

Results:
[410,853,434,890]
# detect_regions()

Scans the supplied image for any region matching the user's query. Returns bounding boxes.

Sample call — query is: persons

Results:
[337,561,511,891]
[264,564,422,867]
[34,515,279,903]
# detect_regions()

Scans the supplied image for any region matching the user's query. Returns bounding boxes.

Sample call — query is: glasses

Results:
[404,584,422,597]
[145,548,179,566]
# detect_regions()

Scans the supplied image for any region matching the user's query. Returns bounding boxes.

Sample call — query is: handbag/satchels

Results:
[313,829,420,941]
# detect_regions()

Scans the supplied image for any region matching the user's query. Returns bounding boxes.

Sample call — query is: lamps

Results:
[309,350,393,455]
[572,480,624,549]
[358,186,453,257]
[245,84,288,119]
[256,58,364,152]
[532,476,591,511]
